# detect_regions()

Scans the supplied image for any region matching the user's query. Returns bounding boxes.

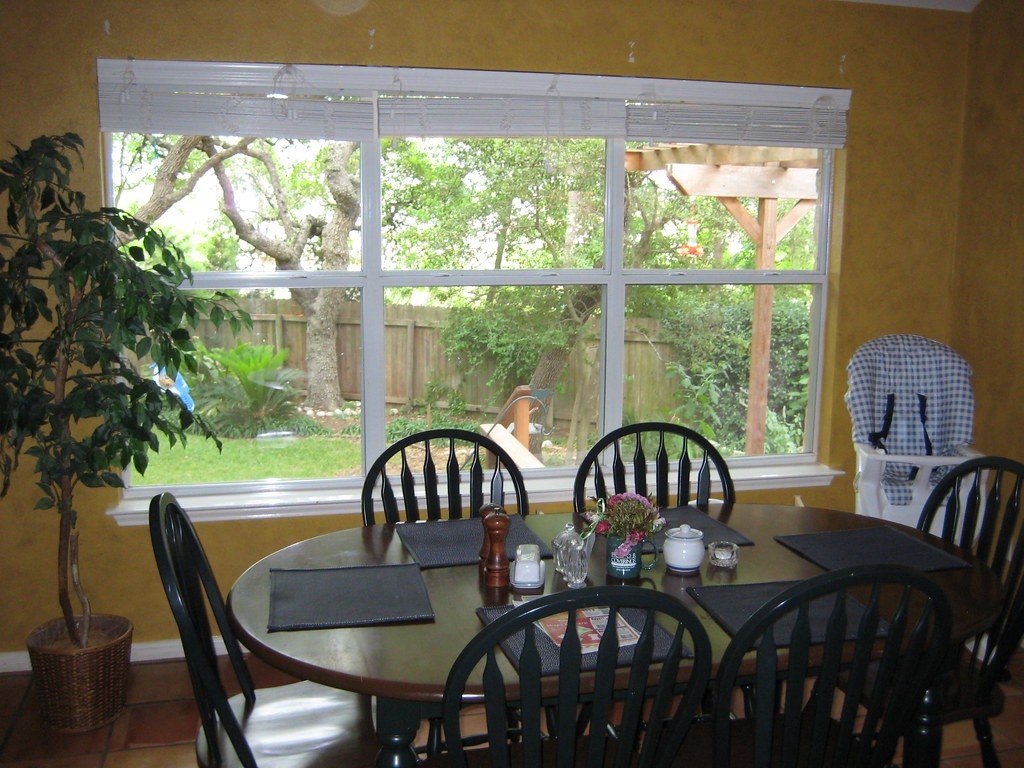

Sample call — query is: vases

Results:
[605,534,658,582]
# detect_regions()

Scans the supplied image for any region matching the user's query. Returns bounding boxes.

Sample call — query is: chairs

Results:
[152,333,1024,768]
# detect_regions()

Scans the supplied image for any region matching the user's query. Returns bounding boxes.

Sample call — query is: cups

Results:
[707,541,739,568]
[551,524,585,573]
[563,540,589,590]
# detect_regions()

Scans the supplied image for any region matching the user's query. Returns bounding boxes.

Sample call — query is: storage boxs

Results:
[509,544,546,589]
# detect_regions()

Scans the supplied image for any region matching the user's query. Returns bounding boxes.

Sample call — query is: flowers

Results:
[580,491,667,558]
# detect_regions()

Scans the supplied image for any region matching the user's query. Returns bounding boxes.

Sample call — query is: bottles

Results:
[663,524,705,572]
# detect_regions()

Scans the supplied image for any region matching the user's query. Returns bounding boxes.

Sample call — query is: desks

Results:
[223,501,1000,768]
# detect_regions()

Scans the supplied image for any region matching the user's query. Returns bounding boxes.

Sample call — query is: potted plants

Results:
[0,132,257,737]
